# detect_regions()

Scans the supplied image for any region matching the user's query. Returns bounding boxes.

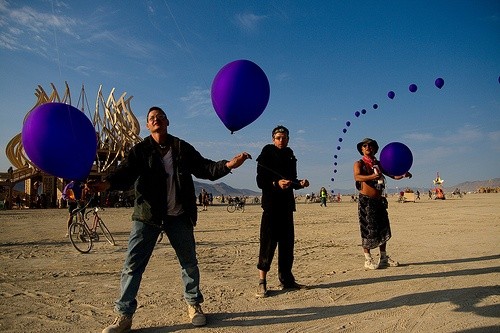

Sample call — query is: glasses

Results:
[147,115,166,121]
[363,143,374,147]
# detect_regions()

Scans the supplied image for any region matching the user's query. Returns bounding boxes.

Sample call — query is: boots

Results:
[102,314,132,333]
[188,303,206,326]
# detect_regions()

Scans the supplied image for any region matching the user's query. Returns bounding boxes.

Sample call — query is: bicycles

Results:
[227,200,245,214]
[68,194,116,254]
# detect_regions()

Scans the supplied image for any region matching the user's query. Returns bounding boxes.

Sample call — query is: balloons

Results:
[388,91,396,99]
[409,84,417,93]
[334,137,343,166]
[343,104,378,133]
[23,102,97,184]
[435,78,444,89]
[331,169,337,193]
[380,142,413,176]
[211,60,270,134]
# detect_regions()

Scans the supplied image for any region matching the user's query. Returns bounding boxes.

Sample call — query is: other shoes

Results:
[255,282,267,299]
[364,258,380,270]
[378,255,399,267]
[280,283,309,290]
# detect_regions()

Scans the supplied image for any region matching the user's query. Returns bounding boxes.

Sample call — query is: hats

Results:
[357,138,379,156]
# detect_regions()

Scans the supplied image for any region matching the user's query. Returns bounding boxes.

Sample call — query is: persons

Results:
[87,107,252,333]
[1,192,67,210]
[294,187,359,208]
[383,188,433,201]
[435,186,500,200]
[195,188,213,211]
[62,181,89,238]
[84,191,136,208]
[256,126,310,297]
[215,193,262,213]
[353,138,412,270]
